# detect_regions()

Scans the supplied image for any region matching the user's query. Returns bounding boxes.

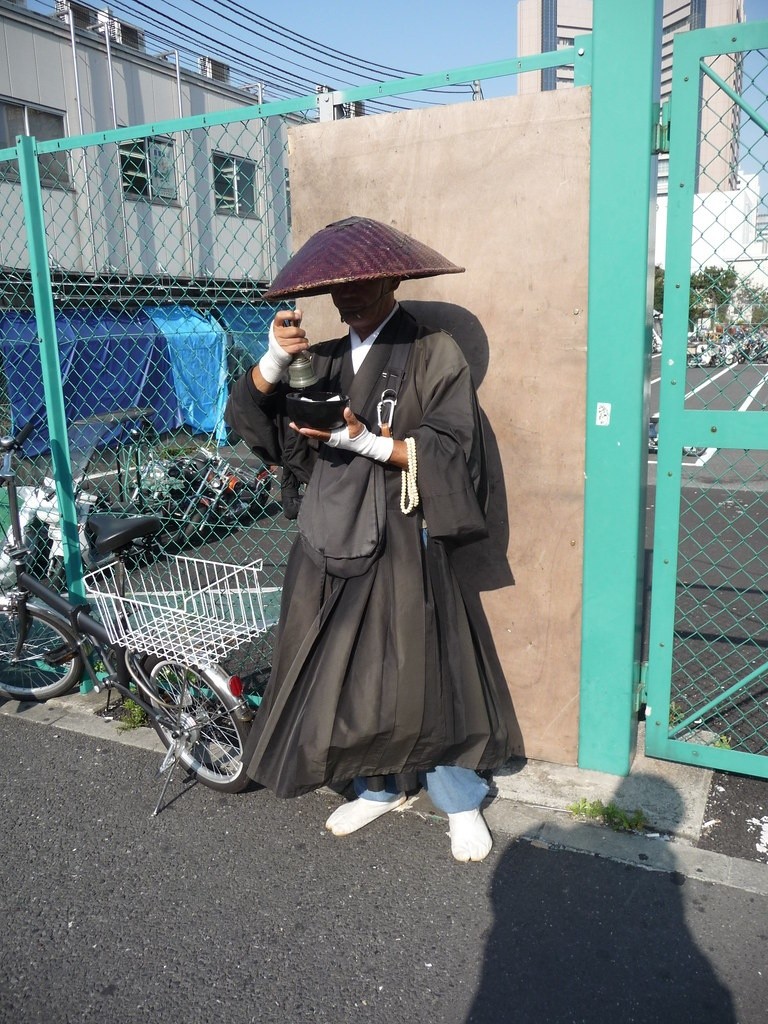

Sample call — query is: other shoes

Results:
[447,807,492,862]
[325,792,407,837]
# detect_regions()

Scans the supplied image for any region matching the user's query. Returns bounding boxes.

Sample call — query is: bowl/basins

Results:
[286,391,351,431]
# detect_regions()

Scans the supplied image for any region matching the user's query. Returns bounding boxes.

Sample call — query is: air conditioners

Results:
[199,56,231,85]
[315,84,366,118]
[111,18,147,53]
[55,1,99,35]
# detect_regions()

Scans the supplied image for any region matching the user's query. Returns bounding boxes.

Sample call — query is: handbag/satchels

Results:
[296,429,386,578]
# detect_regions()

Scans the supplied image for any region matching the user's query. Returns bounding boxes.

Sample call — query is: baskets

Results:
[81,552,269,668]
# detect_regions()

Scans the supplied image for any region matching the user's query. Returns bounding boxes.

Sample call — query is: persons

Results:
[224,217,492,862]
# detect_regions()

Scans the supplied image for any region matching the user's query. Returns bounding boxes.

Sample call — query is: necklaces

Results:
[400,437,419,515]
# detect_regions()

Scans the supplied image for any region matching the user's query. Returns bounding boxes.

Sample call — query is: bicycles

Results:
[0,415,261,818]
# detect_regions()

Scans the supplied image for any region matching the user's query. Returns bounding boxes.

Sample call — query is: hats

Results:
[261,214,467,298]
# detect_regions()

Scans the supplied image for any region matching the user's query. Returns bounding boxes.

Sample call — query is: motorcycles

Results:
[686,328,768,370]
